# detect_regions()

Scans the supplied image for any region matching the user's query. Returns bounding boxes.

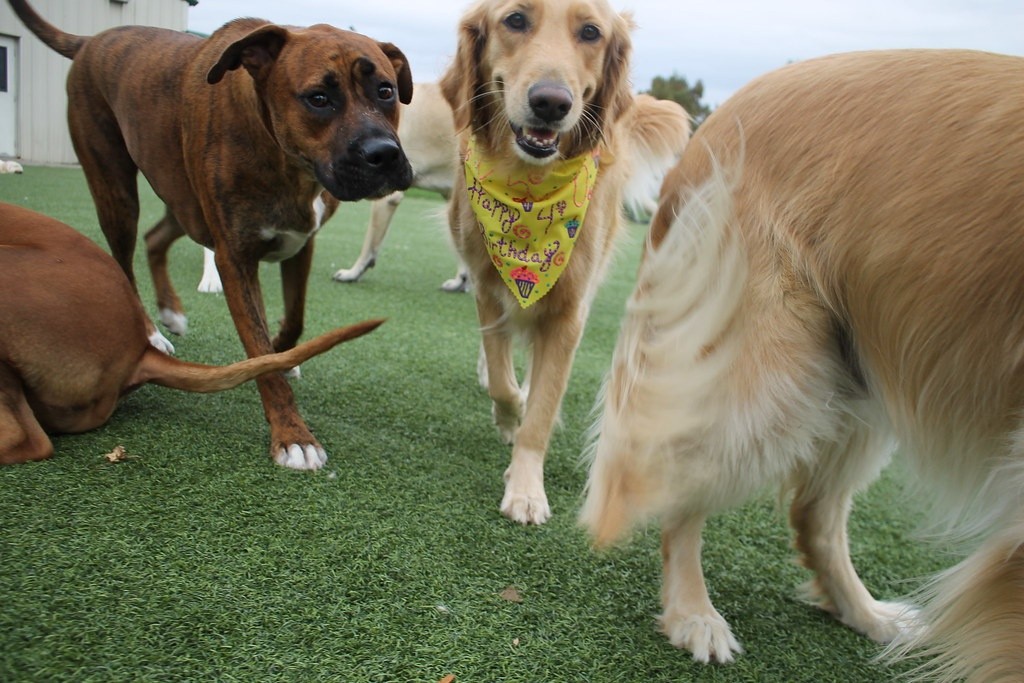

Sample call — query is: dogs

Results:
[437,0,698,528]
[0,2,469,475]
[575,48,1024,683]
